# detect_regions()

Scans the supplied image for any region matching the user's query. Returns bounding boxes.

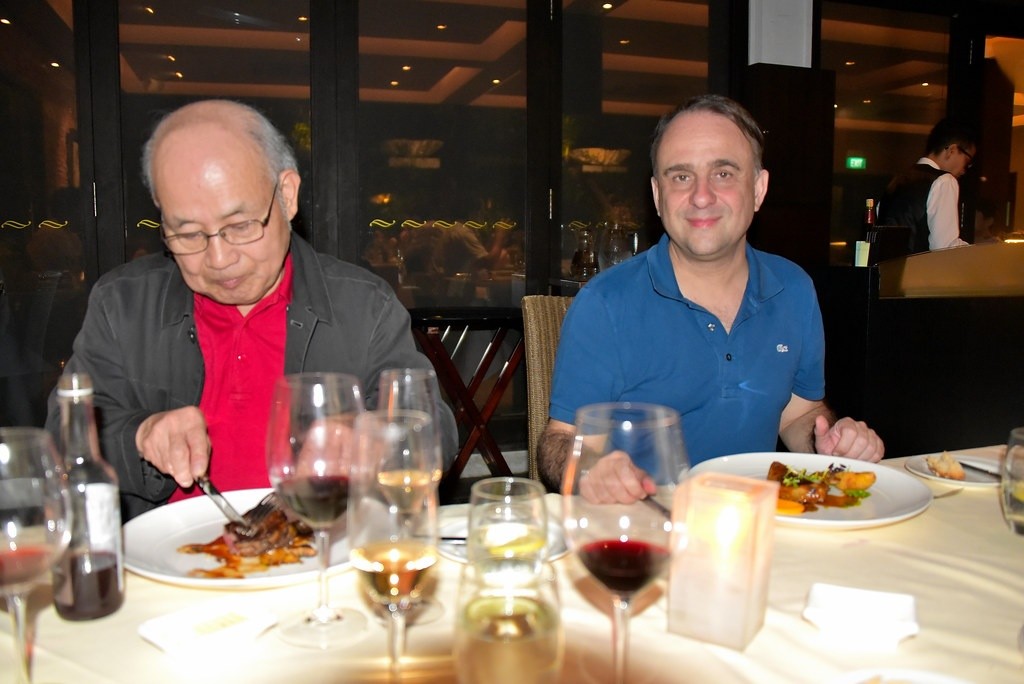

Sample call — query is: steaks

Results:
[224,505,301,557]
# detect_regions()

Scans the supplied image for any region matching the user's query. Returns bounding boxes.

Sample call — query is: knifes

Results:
[193,474,248,525]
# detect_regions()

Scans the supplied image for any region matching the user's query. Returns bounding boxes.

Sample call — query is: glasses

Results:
[945,144,976,169]
[160,170,280,255]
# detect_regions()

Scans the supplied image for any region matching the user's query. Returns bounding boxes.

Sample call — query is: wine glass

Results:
[348,368,443,684]
[561,401,687,683]
[261,373,367,641]
[0,429,75,684]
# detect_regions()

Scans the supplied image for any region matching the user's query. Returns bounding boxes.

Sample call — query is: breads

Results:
[927,449,965,480]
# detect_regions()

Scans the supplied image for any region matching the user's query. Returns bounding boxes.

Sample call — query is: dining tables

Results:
[0,442,1024,683]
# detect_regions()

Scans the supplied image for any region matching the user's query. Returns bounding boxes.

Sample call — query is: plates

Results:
[437,517,567,561]
[905,454,999,487]
[121,488,388,588]
[687,452,933,528]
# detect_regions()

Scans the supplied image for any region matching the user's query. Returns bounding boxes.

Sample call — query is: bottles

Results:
[569,228,595,282]
[864,199,875,224]
[51,372,126,622]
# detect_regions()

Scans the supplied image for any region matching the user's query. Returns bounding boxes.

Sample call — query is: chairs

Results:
[521,295,574,493]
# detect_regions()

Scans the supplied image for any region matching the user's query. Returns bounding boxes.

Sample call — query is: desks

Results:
[412,307,524,501]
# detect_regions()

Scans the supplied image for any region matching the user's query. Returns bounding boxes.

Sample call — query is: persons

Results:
[873,118,978,400]
[43,100,459,526]
[535,94,885,505]
[356,195,524,298]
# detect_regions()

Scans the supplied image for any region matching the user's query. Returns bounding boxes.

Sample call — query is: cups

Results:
[452,557,565,683]
[468,478,548,559]
[998,427,1024,534]
[599,223,629,264]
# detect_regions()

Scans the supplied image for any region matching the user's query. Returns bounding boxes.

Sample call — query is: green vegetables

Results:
[780,463,871,499]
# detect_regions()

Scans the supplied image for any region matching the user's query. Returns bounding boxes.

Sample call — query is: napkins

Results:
[142,594,274,658]
[802,582,917,652]
